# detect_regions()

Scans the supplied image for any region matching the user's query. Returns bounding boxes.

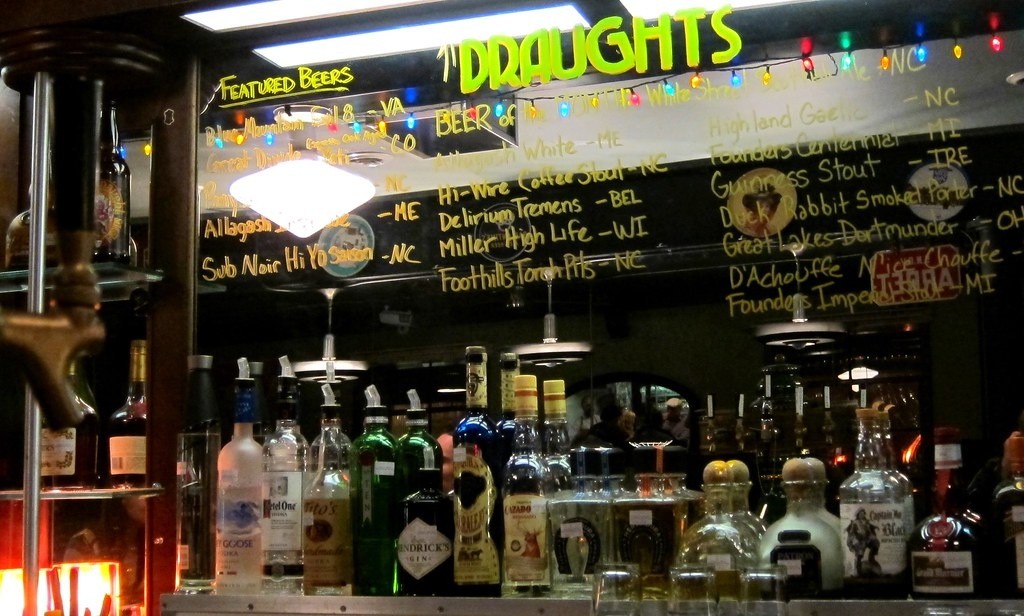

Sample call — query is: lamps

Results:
[229,103,374,237]
[292,286,370,384]
[510,269,591,369]
[755,241,846,351]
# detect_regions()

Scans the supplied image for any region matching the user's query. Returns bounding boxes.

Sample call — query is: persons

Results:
[438,434,453,495]
[570,394,690,446]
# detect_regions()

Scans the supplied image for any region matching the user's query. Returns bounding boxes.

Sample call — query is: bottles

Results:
[40,351,98,488]
[97,101,132,263]
[177,346,1023,598]
[109,340,148,485]
